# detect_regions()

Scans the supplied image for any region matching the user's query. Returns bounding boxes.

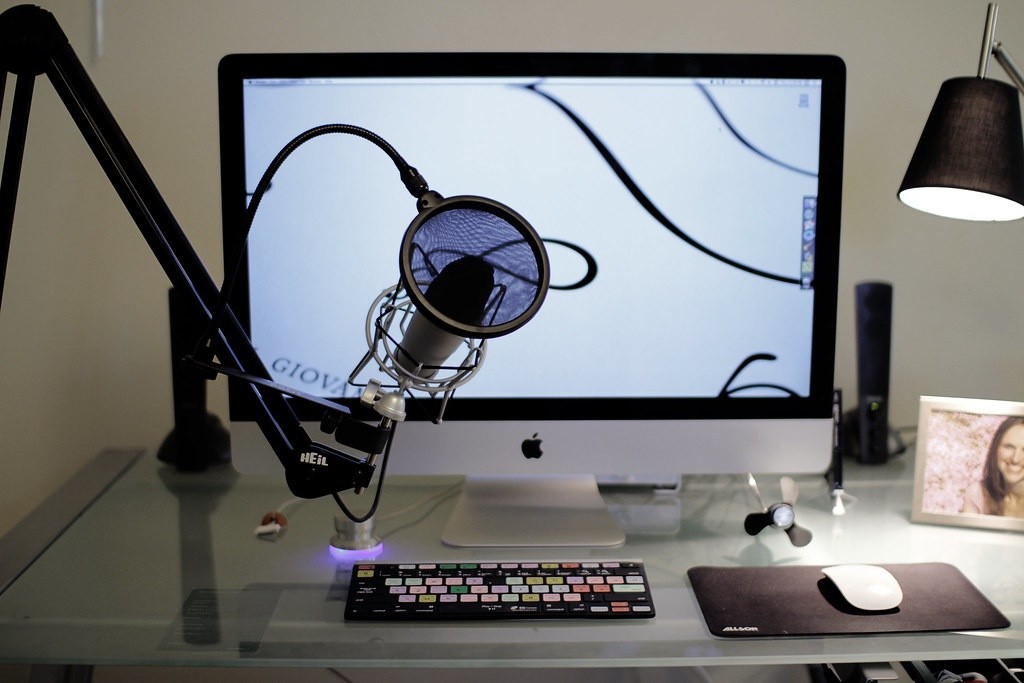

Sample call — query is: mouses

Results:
[821,563,903,611]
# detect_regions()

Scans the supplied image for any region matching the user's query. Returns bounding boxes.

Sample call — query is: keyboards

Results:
[344,561,656,622]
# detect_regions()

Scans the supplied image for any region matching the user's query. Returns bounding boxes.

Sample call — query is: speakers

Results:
[155,289,233,475]
[852,283,898,463]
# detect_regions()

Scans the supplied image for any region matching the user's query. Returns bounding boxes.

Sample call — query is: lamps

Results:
[897,3,1024,223]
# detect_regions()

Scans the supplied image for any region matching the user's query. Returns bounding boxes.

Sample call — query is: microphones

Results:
[392,257,496,384]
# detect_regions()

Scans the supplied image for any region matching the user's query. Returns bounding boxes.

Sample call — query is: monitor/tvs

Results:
[219,51,847,546]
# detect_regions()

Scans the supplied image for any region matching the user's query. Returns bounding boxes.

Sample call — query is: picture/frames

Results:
[909,394,1024,534]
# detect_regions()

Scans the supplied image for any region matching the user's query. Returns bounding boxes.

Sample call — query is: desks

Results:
[0,443,1024,683]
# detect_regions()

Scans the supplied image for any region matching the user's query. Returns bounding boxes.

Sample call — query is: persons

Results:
[959,415,1024,518]
